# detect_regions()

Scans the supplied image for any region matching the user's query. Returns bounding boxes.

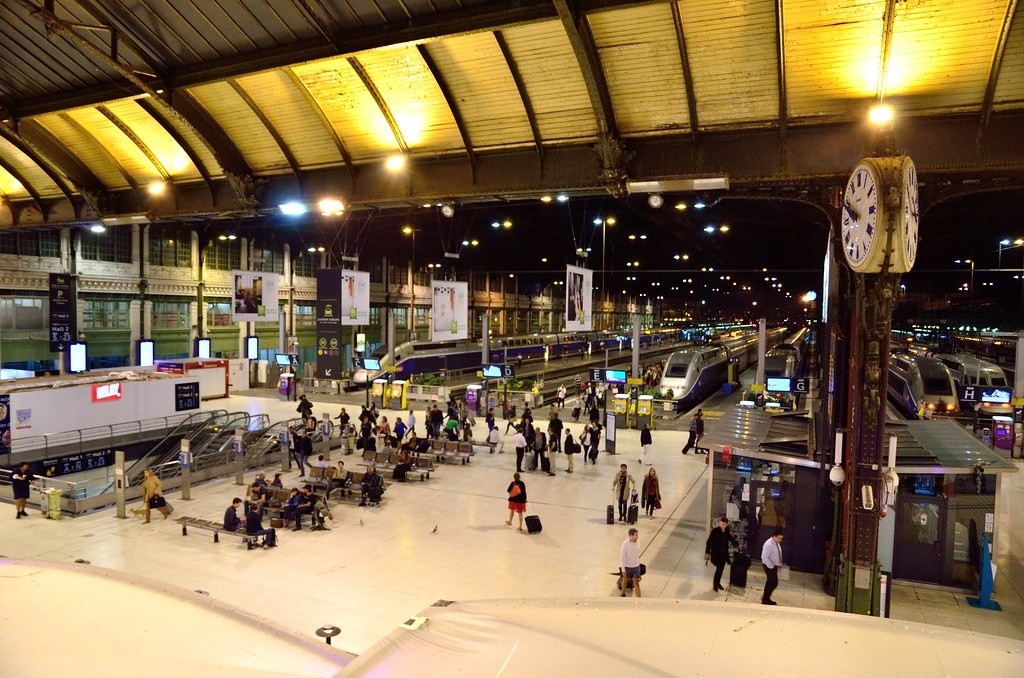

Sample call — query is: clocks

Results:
[901,156,918,272]
[841,163,883,272]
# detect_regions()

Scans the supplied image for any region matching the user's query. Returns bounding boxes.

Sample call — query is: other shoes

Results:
[619,516,622,521]
[714,585,719,592]
[646,510,648,514]
[718,583,725,590]
[762,599,777,605]
[650,515,653,520]
[518,527,523,531]
[16,510,28,519]
[624,515,627,522]
[505,520,512,526]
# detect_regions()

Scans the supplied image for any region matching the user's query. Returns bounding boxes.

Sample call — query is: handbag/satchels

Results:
[655,499,661,509]
[633,490,639,503]
[733,550,752,568]
[509,416,517,423]
[148,494,166,509]
[573,443,581,453]
[509,481,521,497]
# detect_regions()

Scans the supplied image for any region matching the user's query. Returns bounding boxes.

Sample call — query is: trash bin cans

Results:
[40,488,61,519]
[341,435,349,455]
[348,435,354,453]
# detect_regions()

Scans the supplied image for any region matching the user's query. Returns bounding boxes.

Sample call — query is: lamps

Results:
[867,103,897,157]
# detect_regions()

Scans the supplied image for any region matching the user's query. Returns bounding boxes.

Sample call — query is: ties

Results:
[776,543,782,563]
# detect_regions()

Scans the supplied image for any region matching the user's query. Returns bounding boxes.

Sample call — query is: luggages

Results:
[544,457,551,471]
[617,576,642,590]
[730,562,747,587]
[523,445,533,470]
[627,495,638,524]
[607,490,614,524]
[589,448,598,459]
[525,502,542,533]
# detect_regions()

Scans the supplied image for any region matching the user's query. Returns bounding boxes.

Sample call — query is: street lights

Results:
[403,227,421,340]
[594,218,615,338]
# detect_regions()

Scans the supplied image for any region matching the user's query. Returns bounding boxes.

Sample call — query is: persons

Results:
[304,410,317,436]
[358,465,379,506]
[729,477,746,521]
[688,409,708,454]
[485,374,625,476]
[638,423,652,466]
[641,468,662,519]
[620,528,641,597]
[10,462,33,519]
[141,468,162,524]
[704,517,742,592]
[612,464,637,523]
[504,473,526,531]
[288,426,313,477]
[761,490,777,527]
[316,455,327,468]
[761,532,783,605]
[334,396,469,482]
[296,395,313,423]
[626,359,668,392]
[223,470,318,549]
[323,461,347,500]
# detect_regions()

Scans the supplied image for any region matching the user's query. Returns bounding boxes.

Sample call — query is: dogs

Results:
[127,509,146,518]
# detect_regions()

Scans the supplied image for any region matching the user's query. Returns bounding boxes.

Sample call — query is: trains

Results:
[659,327,790,412]
[353,329,676,391]
[887,331,1024,419]
[752,327,812,411]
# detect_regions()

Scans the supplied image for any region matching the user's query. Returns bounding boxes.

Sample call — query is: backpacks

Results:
[619,564,646,575]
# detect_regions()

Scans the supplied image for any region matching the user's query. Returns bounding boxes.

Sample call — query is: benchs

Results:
[172,437,497,550]
